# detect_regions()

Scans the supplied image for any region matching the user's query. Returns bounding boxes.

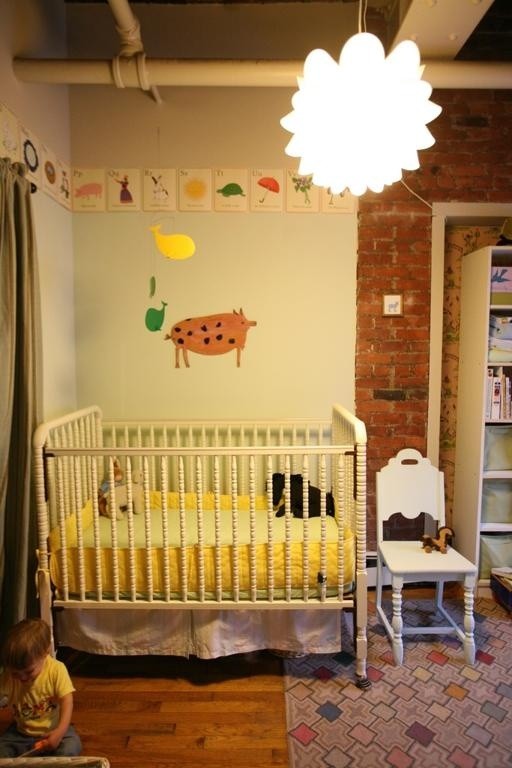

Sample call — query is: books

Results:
[486,266,512,422]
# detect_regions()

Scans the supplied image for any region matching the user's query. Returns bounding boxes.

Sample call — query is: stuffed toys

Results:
[265,473,336,519]
[96,457,147,522]
[421,527,455,554]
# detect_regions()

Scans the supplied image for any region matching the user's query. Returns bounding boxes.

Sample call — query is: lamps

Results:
[278,0,444,196]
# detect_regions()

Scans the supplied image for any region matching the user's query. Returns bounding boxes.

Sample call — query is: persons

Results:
[0,617,82,758]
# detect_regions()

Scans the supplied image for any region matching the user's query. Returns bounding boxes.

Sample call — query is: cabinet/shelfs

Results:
[452,246,512,598]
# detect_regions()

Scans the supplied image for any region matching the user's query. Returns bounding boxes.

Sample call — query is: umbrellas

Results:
[257,176,279,203]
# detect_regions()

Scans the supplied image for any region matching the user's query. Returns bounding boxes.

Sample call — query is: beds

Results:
[32,405,368,683]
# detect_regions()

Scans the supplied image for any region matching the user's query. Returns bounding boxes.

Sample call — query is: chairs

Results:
[375,449,478,668]
